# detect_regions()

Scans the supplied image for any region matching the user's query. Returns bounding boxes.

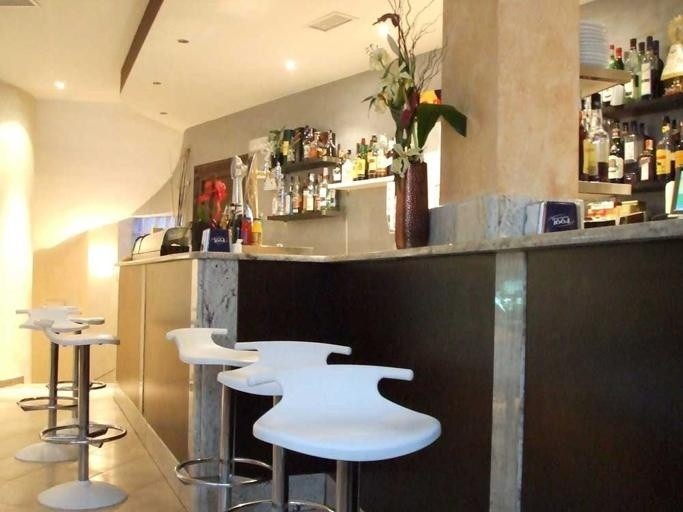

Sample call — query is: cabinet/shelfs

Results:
[577,60,682,199]
[265,156,341,222]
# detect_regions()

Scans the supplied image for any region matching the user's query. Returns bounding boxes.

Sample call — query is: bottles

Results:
[578,35,682,185]
[225,204,262,247]
[267,125,396,216]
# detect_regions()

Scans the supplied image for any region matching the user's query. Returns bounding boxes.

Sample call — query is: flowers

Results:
[360,0,471,199]
[190,178,228,227]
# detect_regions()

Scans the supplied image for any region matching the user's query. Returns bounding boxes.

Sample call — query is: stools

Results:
[14,303,127,508]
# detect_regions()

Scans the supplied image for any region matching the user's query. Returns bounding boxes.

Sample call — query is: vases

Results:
[394,162,429,248]
[190,221,206,251]
